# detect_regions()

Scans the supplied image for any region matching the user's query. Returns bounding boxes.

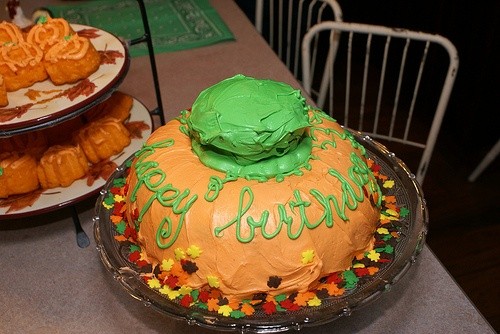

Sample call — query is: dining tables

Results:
[0,0,500,334]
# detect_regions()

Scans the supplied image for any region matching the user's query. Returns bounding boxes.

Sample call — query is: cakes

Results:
[103,74,409,319]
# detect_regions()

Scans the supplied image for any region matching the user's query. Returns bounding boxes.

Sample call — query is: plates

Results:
[93,126,429,334]
[0,21,131,138]
[0,92,155,219]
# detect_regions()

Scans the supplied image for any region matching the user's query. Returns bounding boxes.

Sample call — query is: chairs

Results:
[302,21,459,188]
[256,0,342,110]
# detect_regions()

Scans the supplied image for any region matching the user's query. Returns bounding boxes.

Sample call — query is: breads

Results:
[0,92,133,199]
[0,17,101,107]
